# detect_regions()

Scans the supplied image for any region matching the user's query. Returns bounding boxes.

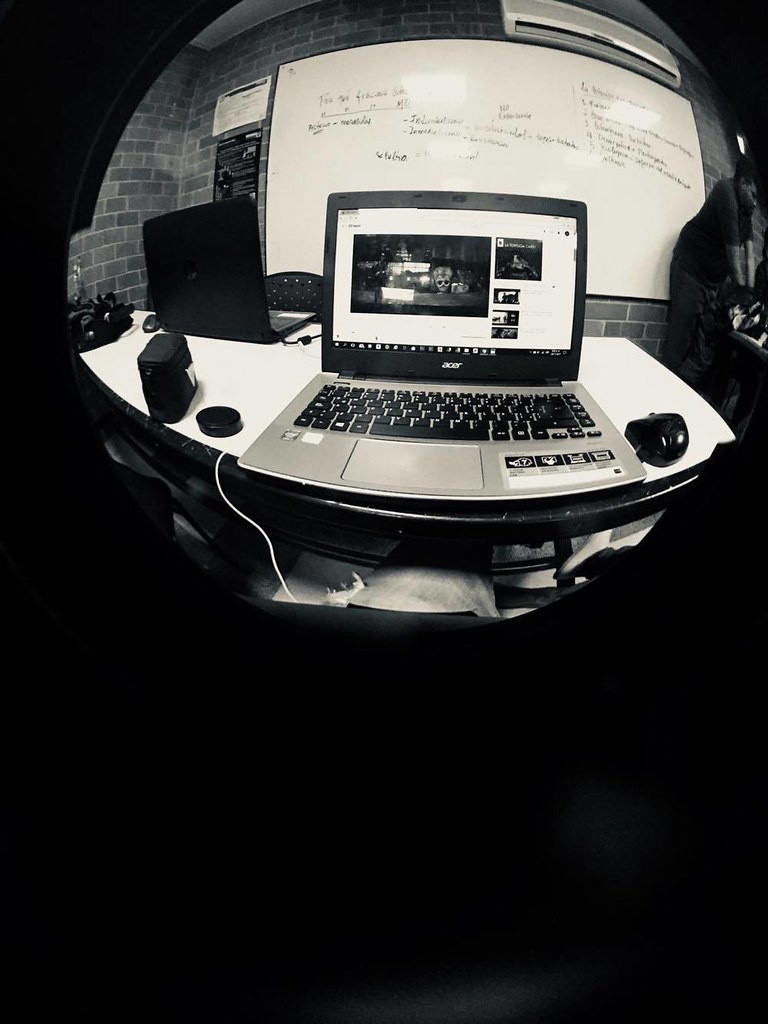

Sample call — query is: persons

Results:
[664,157,759,392]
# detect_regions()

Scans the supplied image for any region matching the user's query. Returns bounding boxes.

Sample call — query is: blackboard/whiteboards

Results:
[262,35,707,306]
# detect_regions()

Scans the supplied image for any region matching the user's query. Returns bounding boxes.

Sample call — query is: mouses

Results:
[625,412,688,468]
[141,314,159,333]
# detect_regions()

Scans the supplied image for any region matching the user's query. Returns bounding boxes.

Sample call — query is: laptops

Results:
[144,192,317,345]
[237,190,646,502]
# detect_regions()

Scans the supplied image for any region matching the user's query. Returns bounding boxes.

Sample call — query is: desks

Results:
[79,307,736,623]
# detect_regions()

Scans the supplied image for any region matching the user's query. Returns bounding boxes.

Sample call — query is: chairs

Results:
[264,271,323,324]
